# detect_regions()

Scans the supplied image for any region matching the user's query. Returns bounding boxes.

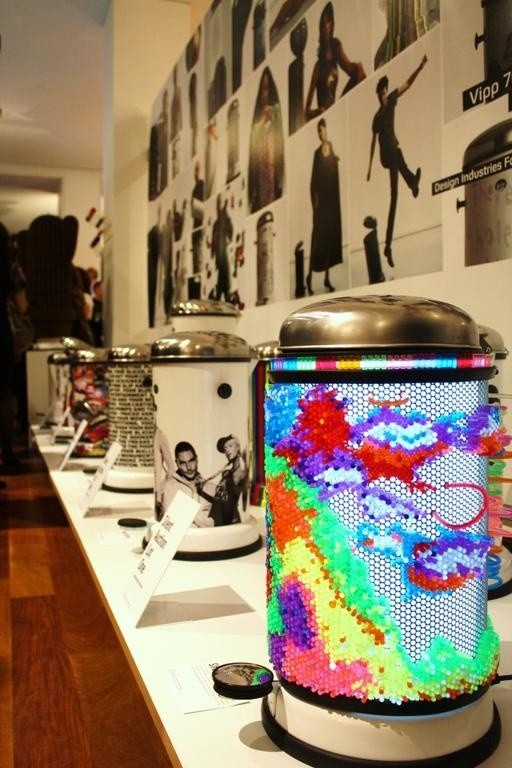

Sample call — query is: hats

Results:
[217,435,234,454]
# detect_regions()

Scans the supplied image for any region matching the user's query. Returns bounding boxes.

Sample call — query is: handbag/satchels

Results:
[6,255,37,361]
[68,262,95,347]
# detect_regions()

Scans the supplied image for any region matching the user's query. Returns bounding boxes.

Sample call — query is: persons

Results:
[367,56,428,269]
[246,68,284,208]
[208,194,240,302]
[162,442,214,528]
[305,117,342,294]
[208,433,248,525]
[147,200,189,328]
[191,159,207,273]
[154,394,176,517]
[304,0,365,114]
